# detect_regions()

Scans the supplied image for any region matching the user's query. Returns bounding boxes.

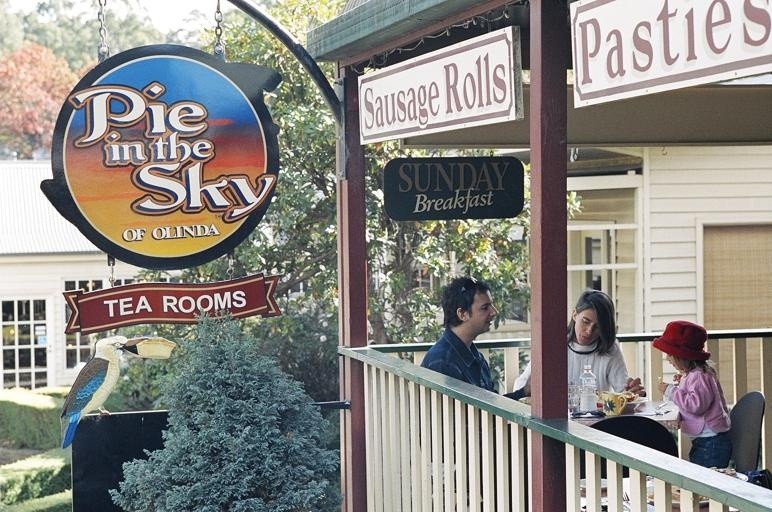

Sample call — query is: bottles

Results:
[579,365,597,412]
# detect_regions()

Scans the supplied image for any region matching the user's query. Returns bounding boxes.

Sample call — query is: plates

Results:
[518,396,531,405]
[625,396,649,411]
[648,472,749,508]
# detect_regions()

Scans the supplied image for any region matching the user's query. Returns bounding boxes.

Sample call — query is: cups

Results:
[568,385,579,411]
[602,393,627,416]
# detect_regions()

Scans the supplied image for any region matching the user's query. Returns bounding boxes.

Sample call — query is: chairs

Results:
[730,391,766,473]
[582,415,679,479]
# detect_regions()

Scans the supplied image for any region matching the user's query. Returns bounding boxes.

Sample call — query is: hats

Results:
[653,321,710,360]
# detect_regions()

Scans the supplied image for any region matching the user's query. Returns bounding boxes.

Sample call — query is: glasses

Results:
[461,276,478,292]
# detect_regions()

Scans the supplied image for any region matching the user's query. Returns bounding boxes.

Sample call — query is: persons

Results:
[512,289,647,397]
[410,277,528,512]
[653,320,733,469]
